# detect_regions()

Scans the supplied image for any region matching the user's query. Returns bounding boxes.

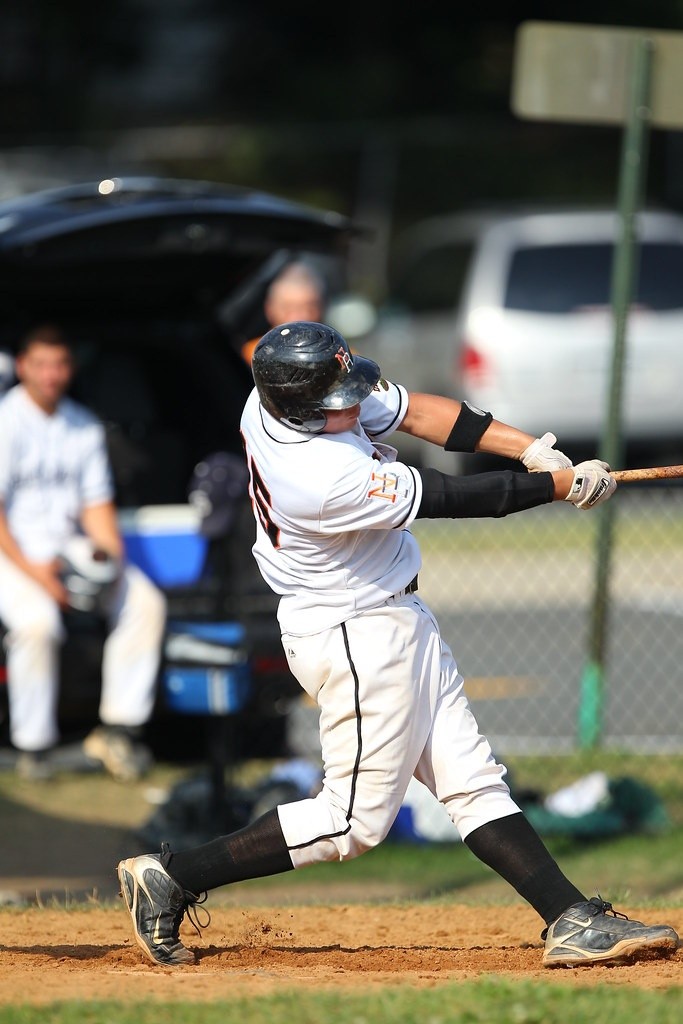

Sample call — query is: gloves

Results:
[522,431,571,474]
[564,460,616,509]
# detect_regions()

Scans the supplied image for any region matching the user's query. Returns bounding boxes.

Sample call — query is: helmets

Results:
[252,320,384,432]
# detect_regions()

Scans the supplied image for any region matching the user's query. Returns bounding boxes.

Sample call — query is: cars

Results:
[1,174,364,725]
[362,196,681,481]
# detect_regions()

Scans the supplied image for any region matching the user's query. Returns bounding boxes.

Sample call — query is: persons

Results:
[238,264,362,376]
[116,321,678,967]
[0,328,170,786]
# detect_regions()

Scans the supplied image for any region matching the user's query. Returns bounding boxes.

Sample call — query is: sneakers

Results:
[118,840,199,967]
[540,897,678,968]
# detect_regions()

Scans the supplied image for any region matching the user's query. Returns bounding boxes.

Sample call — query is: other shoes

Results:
[15,726,145,783]
